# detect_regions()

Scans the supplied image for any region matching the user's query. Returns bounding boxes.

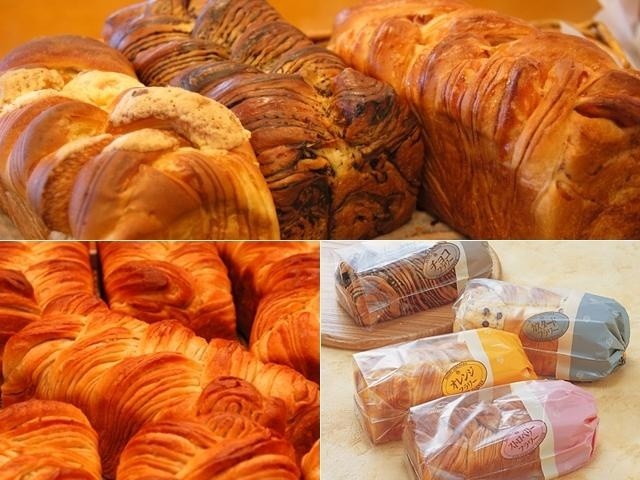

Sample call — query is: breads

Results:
[1,243,320,480]
[337,245,557,480]
[0,0,640,239]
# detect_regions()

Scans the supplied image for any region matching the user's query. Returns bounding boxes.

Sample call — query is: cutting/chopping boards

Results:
[320,239,505,353]
